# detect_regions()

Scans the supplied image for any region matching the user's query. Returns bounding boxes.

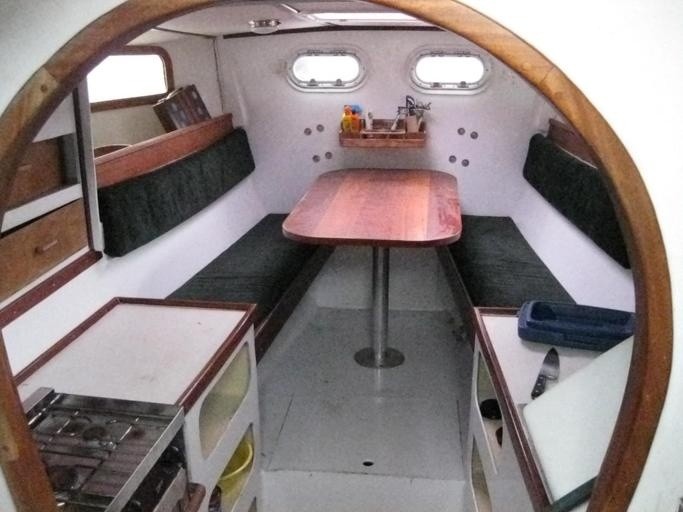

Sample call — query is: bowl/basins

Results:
[218,438,254,494]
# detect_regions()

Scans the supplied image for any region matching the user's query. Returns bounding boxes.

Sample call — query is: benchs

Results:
[431,214,579,355]
[163,213,335,368]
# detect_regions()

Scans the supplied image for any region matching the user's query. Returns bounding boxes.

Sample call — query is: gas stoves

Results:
[21,387,185,511]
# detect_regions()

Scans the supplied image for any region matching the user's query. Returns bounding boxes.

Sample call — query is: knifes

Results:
[531,347,561,399]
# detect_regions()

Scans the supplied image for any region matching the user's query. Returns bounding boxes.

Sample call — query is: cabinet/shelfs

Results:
[186,325,263,512]
[0,103,93,304]
[464,330,535,512]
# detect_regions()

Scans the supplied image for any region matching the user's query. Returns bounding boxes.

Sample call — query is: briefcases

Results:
[152,84,212,134]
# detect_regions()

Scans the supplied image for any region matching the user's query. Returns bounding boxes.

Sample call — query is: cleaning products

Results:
[340,105,373,133]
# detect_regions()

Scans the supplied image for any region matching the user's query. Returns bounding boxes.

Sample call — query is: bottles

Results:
[342,104,373,133]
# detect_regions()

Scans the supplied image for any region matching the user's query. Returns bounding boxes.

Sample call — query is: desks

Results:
[283,162,463,366]
